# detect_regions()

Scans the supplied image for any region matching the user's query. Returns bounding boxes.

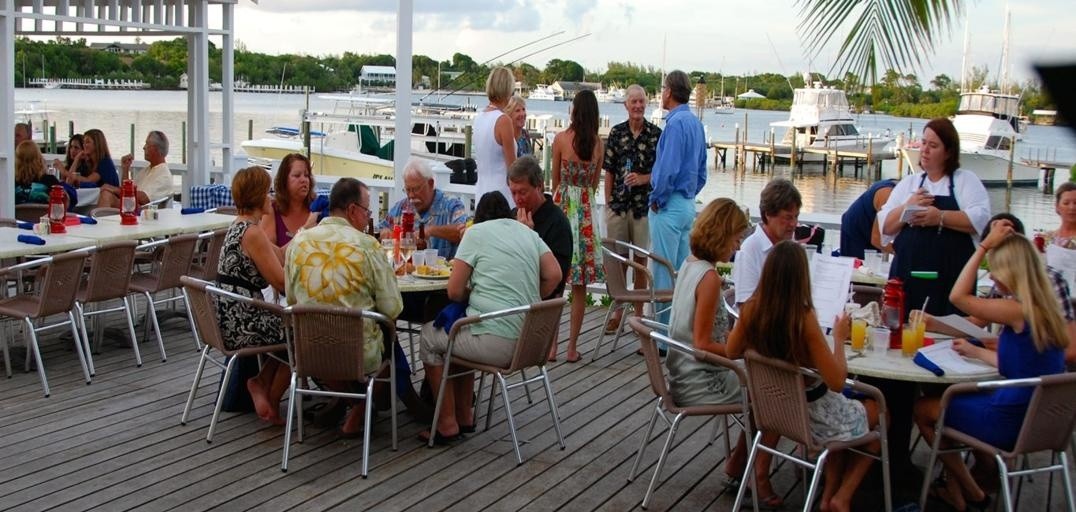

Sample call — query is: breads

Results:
[416,266,432,275]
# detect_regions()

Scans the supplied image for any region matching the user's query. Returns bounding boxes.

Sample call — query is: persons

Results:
[506,160,573,301]
[58,133,85,181]
[733,179,822,476]
[875,114,993,326]
[54,128,120,187]
[472,65,520,219]
[634,69,709,360]
[726,240,892,511]
[215,166,291,424]
[669,200,784,511]
[545,88,606,364]
[15,122,33,153]
[272,156,332,399]
[419,191,562,443]
[15,139,61,206]
[914,220,1070,512]
[286,179,420,438]
[502,96,535,162]
[598,82,667,336]
[840,179,902,259]
[95,130,174,209]
[909,213,1076,490]
[374,161,466,260]
[1034,184,1076,372]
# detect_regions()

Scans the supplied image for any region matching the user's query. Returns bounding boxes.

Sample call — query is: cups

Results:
[424,248,438,264]
[868,252,882,271]
[871,327,889,360]
[847,302,860,316]
[900,323,916,358]
[908,316,927,349]
[69,170,82,189]
[850,318,865,353]
[411,249,424,266]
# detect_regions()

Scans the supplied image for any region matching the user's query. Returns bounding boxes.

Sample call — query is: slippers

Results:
[419,424,476,441]
[303,402,363,439]
[720,481,778,509]
[930,479,990,512]
[567,351,580,362]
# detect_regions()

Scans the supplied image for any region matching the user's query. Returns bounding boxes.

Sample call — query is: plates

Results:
[411,270,449,279]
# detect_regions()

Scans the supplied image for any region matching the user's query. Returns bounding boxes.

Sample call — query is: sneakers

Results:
[605,319,624,334]
[637,347,666,357]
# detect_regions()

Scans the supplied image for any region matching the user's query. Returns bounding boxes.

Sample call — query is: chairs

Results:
[399,309,434,373]
[130,233,201,363]
[12,196,239,220]
[715,218,1012,340]
[74,241,142,377]
[626,316,753,510]
[281,303,397,480]
[179,276,304,445]
[733,344,891,512]
[2,248,92,396]
[591,239,677,359]
[428,298,566,463]
[919,372,1074,511]
[190,228,227,282]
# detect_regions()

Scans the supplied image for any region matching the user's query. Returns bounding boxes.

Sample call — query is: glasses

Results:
[402,181,427,194]
[354,203,372,217]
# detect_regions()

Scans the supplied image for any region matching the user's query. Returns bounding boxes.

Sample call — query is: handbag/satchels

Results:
[215,356,258,412]
[795,224,824,253]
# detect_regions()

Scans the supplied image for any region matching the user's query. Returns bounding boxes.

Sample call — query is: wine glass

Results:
[397,237,416,283]
[380,239,394,264]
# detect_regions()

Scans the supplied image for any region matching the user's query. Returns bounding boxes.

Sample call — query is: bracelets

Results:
[979,243,989,253]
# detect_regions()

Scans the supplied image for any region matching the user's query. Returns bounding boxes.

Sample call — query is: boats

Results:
[900,91,1046,185]
[14,100,69,163]
[753,88,894,164]
[42,80,62,89]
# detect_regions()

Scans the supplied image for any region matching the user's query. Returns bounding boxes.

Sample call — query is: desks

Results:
[378,244,448,293]
[2,209,240,259]
[820,325,1005,383]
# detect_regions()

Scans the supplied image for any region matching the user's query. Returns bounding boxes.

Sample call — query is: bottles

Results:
[38,215,51,236]
[118,177,140,227]
[400,205,414,234]
[881,276,904,352]
[47,184,68,232]
[416,222,427,250]
[625,157,632,192]
